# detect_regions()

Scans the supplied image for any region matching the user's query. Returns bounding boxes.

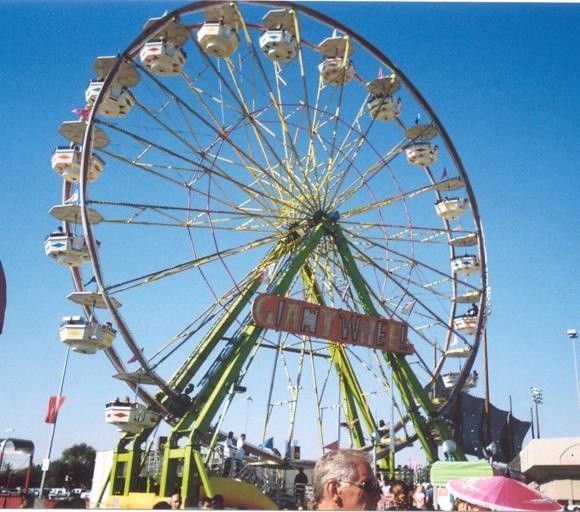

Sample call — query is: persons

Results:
[96,74,105,83]
[222,431,246,478]
[473,370,478,381]
[267,467,308,506]
[313,449,378,511]
[220,15,225,26]
[157,34,166,42]
[68,141,75,150]
[375,91,383,98]
[564,503,569,510]
[365,475,491,511]
[466,302,478,317]
[152,492,182,510]
[201,495,225,510]
[229,25,240,41]
[573,507,579,510]
[275,23,283,30]
[52,227,62,232]
[445,196,449,202]
[107,322,112,328]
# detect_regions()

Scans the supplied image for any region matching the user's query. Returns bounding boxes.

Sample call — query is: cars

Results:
[0,484,90,501]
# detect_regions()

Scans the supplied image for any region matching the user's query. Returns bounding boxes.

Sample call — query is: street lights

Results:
[565,329,580,399]
[529,385,545,439]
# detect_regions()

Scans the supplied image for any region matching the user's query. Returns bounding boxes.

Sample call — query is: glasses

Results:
[343,478,384,493]
